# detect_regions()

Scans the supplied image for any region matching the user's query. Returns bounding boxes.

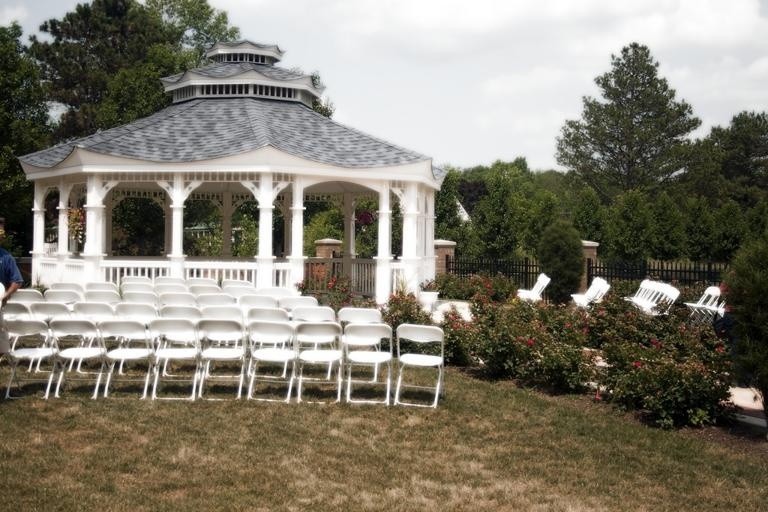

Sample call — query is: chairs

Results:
[515,272,728,324]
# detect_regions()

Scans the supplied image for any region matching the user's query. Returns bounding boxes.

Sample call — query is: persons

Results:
[0,218,24,358]
